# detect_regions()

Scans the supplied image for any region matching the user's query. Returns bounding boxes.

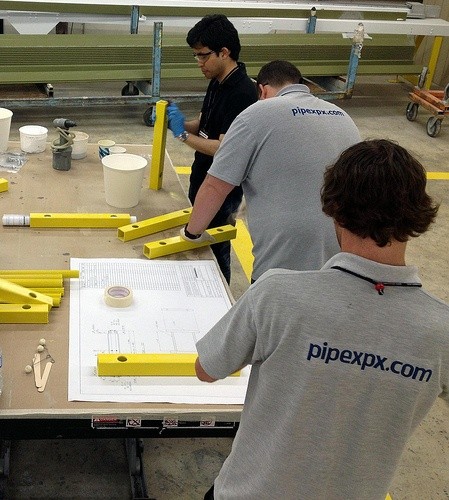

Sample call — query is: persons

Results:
[194,139,449,500]
[179,59,362,284]
[152,14,259,286]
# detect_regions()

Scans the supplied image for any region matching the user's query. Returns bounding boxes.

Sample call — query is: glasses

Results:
[192,50,222,63]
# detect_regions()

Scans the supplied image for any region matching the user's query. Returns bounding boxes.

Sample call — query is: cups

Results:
[98,140,115,160]
[0,107,13,152]
[109,147,127,155]
[69,131,89,160]
[101,154,148,208]
[19,125,48,153]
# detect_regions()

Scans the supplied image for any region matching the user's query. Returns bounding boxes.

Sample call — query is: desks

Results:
[0,141,244,440]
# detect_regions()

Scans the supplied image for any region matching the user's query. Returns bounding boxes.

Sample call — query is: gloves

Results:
[167,102,186,138]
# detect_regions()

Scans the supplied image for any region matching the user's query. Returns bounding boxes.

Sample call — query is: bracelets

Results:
[184,223,201,239]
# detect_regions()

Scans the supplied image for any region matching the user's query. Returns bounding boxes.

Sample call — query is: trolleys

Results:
[405,66,449,138]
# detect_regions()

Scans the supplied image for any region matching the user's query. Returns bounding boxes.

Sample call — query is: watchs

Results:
[179,131,189,141]
